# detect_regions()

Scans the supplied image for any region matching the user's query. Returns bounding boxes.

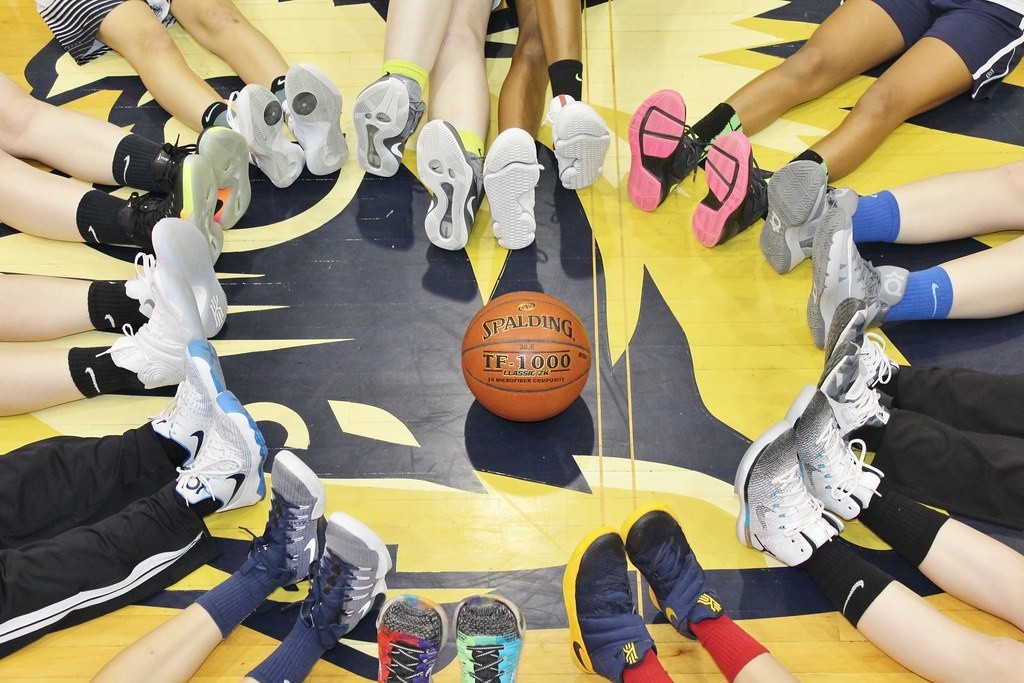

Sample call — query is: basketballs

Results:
[460,289,593,421]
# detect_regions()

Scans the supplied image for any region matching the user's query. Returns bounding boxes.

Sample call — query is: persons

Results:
[626,1,1024,248]
[816,298,1024,528]
[0,74,252,268]
[759,160,1024,351]
[733,384,1024,683]
[36,0,349,188]
[562,505,800,683]
[352,0,502,251]
[89,450,393,683]
[375,594,526,683]
[0,339,269,660]
[0,218,228,417]
[483,0,612,250]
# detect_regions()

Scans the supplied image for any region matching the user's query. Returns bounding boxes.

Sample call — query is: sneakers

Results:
[112,66,899,683]
